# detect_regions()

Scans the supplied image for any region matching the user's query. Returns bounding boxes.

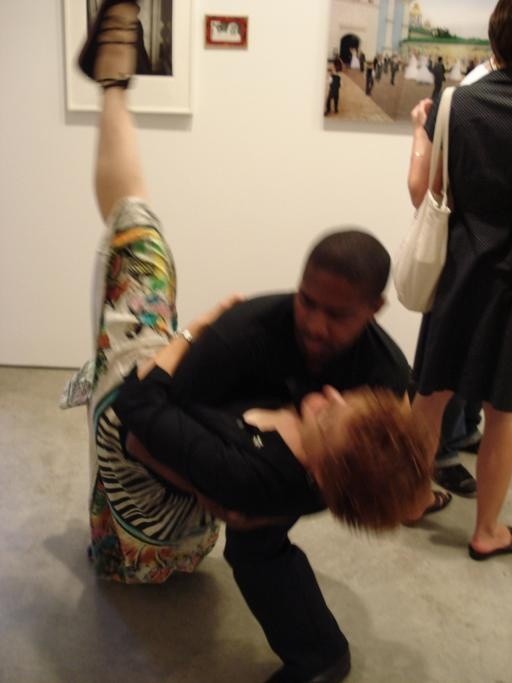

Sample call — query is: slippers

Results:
[431,464,479,499]
[425,488,452,514]
[469,526,512,561]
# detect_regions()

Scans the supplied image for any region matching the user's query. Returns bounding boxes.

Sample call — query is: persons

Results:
[330,46,487,98]
[323,69,341,115]
[400,1,511,560]
[432,57,447,99]
[366,61,375,96]
[58,1,429,584]
[125,231,412,680]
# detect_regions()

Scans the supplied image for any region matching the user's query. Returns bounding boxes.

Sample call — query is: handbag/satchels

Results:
[393,87,451,314]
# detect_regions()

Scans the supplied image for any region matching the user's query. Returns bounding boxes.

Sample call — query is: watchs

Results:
[176,327,197,345]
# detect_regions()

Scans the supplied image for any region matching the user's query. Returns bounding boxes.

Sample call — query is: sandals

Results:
[78,0,142,86]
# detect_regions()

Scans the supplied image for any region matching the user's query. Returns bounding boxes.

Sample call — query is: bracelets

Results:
[408,151,429,163]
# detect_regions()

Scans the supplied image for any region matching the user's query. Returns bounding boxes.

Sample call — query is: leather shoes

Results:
[264,653,349,682]
